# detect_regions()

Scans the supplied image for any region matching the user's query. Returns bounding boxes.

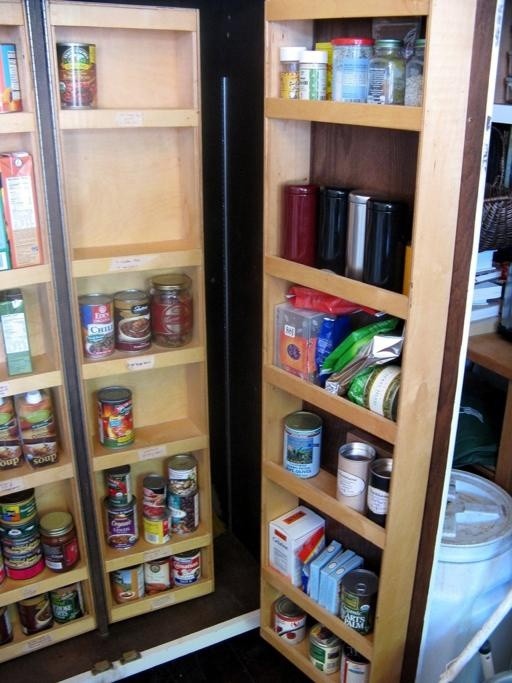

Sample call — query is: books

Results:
[469,247,503,324]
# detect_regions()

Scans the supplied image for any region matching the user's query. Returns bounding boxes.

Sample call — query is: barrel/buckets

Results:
[415,470,511,682]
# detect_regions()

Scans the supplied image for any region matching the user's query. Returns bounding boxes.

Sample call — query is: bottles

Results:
[404,38,424,106]
[297,49,328,101]
[278,44,307,100]
[367,38,404,104]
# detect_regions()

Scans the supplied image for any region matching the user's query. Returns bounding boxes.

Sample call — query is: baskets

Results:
[477,124,512,254]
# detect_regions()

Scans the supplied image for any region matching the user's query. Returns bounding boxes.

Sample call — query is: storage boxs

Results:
[267,503,325,589]
[270,301,326,387]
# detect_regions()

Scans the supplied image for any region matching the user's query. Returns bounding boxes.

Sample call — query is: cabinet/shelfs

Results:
[38,0,220,628]
[0,0,100,670]
[254,0,512,682]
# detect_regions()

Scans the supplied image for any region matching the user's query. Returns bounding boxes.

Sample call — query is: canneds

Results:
[0,487,84,646]
[113,288,151,353]
[272,598,370,683]
[58,42,97,110]
[101,453,202,605]
[77,293,114,361]
[147,273,194,348]
[339,569,379,636]
[95,385,135,448]
